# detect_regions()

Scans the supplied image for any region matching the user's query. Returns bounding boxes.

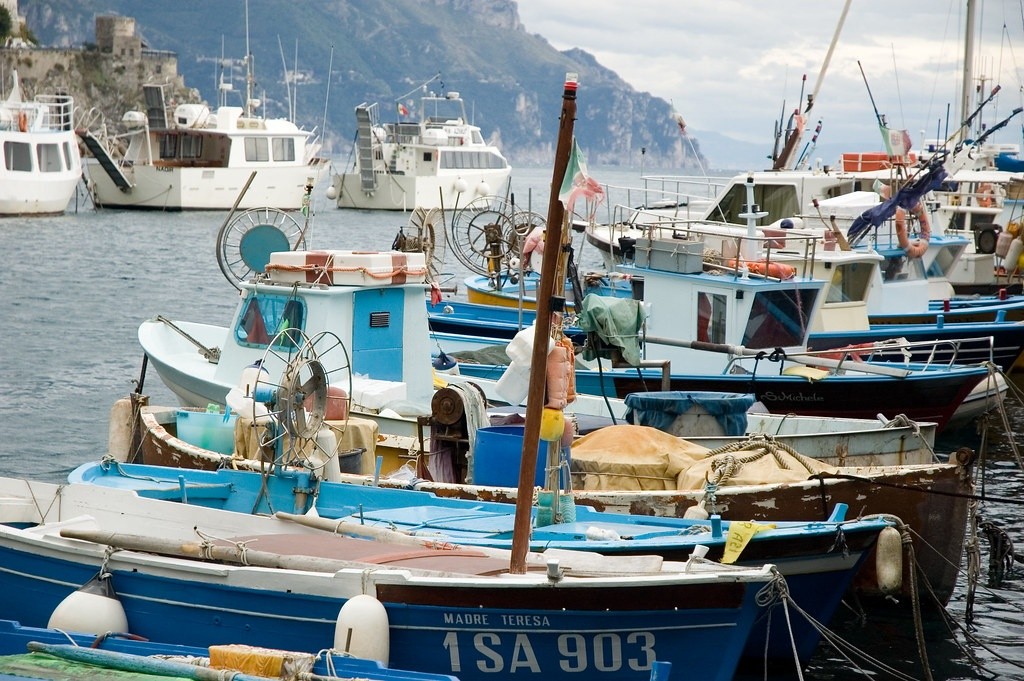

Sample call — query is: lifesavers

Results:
[894,198,931,258]
[975,185,993,206]
[768,262,798,280]
[18,112,27,132]
[726,260,778,277]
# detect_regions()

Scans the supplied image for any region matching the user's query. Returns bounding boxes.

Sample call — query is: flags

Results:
[558,140,605,212]
[873,179,891,200]
[395,101,410,118]
[882,126,914,159]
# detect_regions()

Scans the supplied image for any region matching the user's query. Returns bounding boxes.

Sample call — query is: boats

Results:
[0,0,1024,681]
